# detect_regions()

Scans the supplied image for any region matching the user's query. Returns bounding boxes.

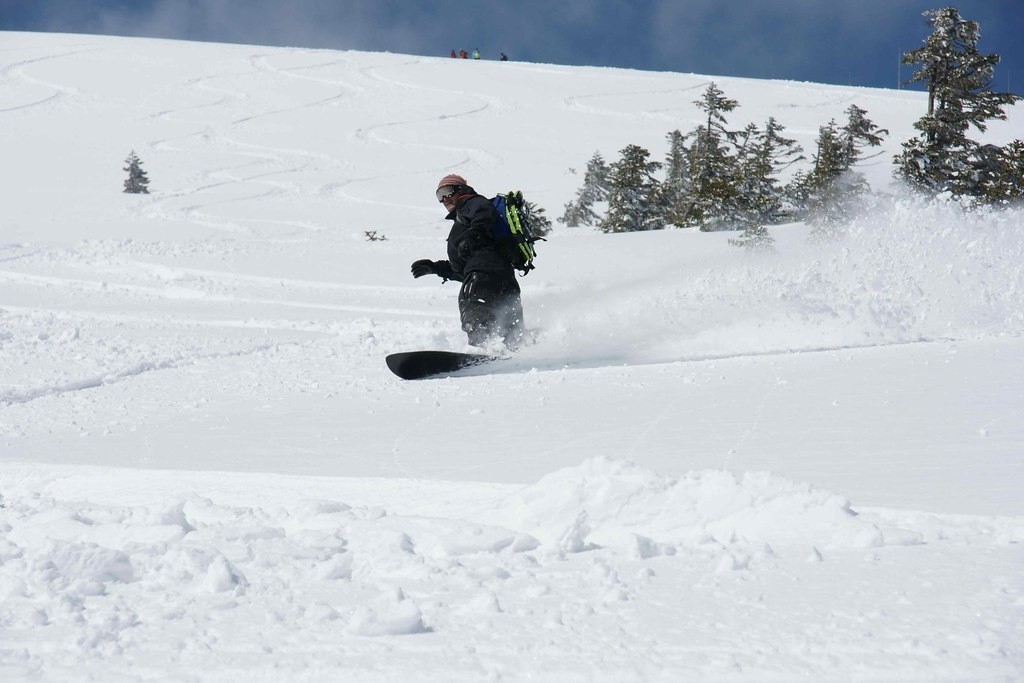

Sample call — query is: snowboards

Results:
[385,350,496,379]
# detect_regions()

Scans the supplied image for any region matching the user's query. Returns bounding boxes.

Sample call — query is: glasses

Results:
[436,185,467,203]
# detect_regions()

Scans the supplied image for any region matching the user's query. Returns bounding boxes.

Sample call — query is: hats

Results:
[438,174,467,186]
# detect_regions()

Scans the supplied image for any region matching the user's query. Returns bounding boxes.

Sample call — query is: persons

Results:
[450,49,457,59]
[501,52,509,63]
[460,49,470,60]
[473,47,482,61]
[411,175,528,354]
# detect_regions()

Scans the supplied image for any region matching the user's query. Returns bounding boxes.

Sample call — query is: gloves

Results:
[449,249,468,273]
[411,259,437,278]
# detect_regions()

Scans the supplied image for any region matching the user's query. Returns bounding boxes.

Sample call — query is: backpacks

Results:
[455,190,547,276]
